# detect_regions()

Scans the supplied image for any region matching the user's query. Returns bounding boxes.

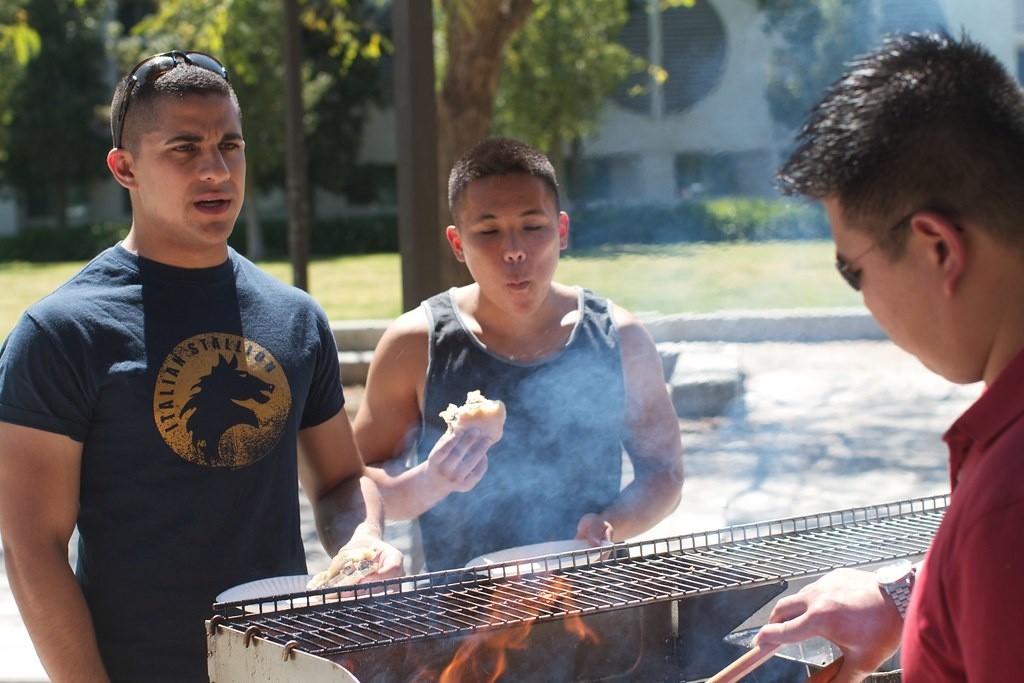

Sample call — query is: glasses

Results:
[117,50,229,149]
[835,208,963,290]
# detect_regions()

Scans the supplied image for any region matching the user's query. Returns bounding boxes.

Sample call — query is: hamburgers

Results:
[438,389,507,446]
[305,546,378,599]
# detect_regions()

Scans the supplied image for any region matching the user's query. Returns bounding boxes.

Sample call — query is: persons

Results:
[351,138,685,590]
[0,49,406,683]
[751,30,1024,683]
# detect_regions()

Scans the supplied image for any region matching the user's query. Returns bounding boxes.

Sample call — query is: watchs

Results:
[875,558,916,621]
